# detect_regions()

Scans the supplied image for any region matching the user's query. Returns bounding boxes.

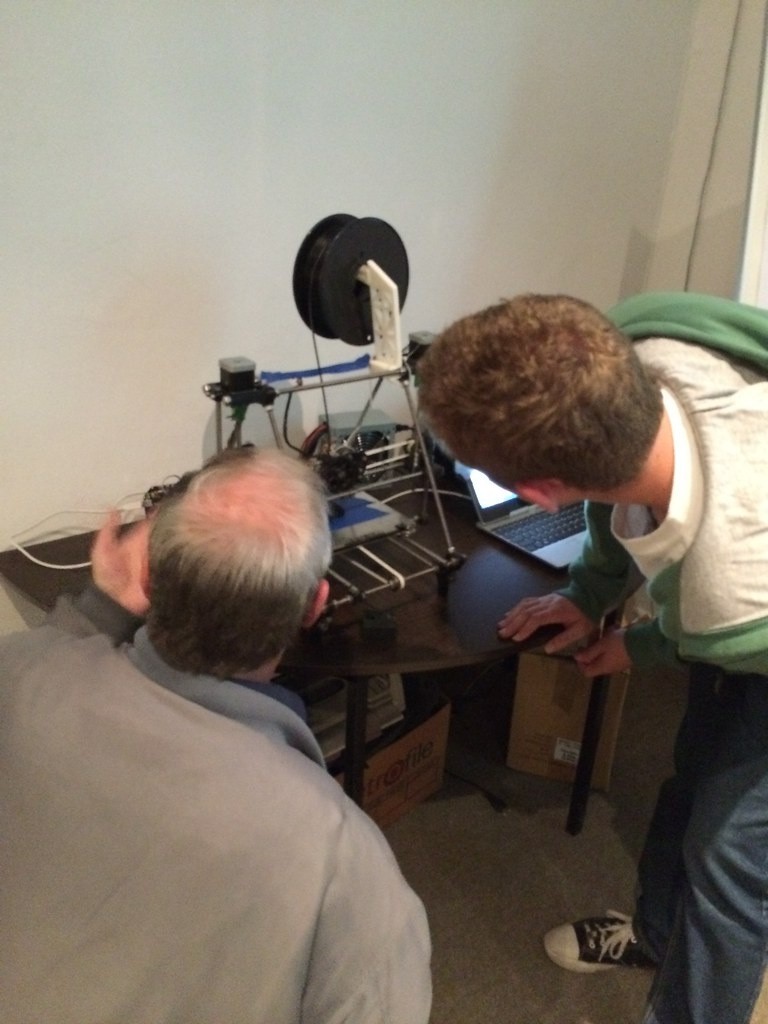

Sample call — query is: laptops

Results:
[462,465,587,568]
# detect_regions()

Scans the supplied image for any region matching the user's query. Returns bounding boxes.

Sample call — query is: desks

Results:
[0,479,647,837]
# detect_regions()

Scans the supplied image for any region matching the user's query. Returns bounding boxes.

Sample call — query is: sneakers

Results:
[543,910,654,972]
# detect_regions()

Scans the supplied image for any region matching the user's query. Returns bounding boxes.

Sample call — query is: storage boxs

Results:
[329,691,452,828]
[505,645,631,793]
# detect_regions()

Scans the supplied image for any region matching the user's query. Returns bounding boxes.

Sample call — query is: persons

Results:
[417,292,768,1024]
[0,450,432,1024]
